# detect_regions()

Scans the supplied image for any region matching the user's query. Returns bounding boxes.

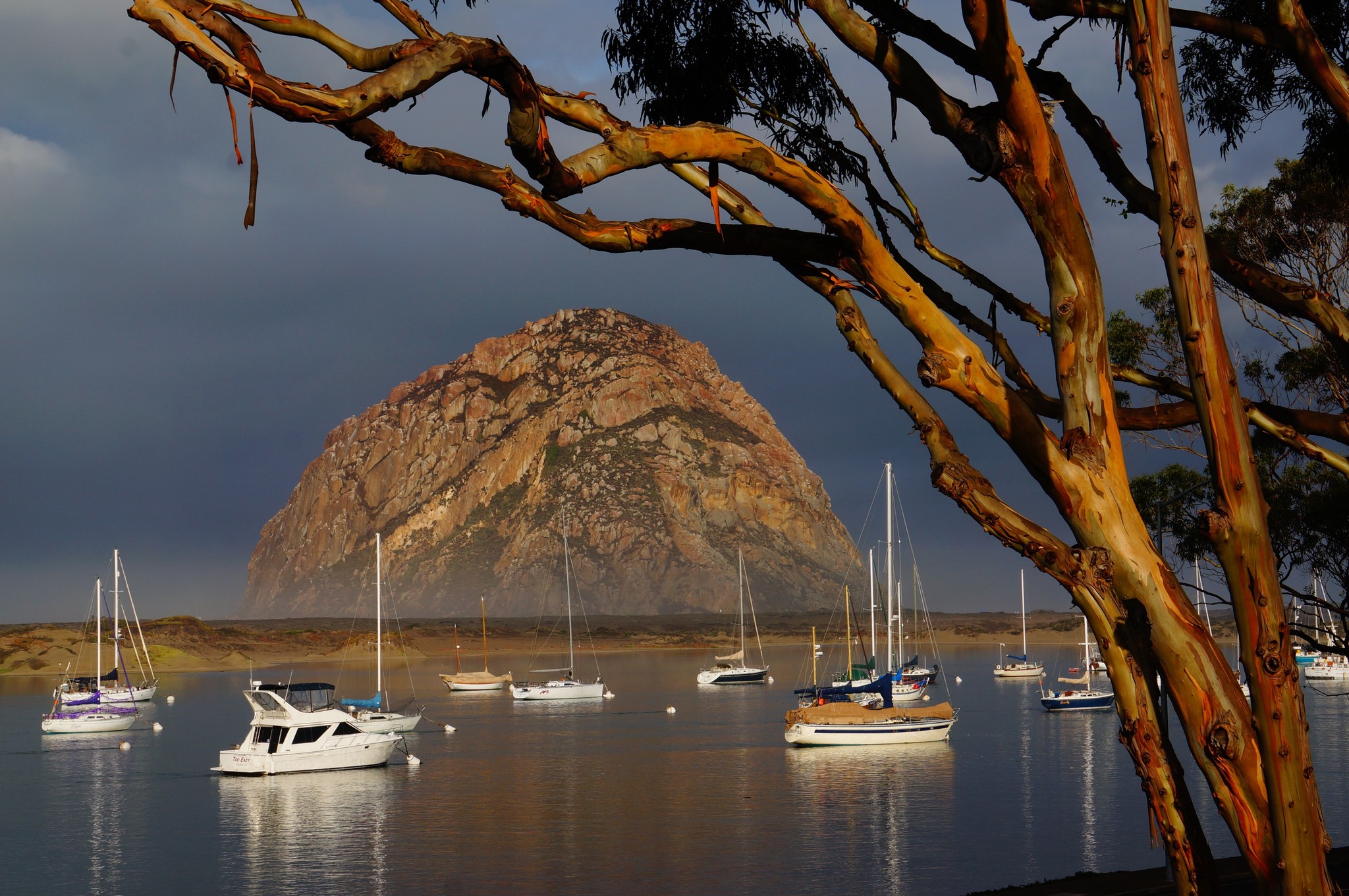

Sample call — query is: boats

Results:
[210,656,403,778]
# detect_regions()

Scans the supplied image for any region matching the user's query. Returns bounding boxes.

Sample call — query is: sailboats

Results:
[441,596,511,691]
[1156,554,1252,710]
[335,531,425,733]
[53,545,162,703]
[1288,565,1349,680]
[696,549,770,685]
[785,458,960,747]
[508,505,604,700]
[1034,613,1116,713]
[40,576,146,733]
[994,569,1044,677]
